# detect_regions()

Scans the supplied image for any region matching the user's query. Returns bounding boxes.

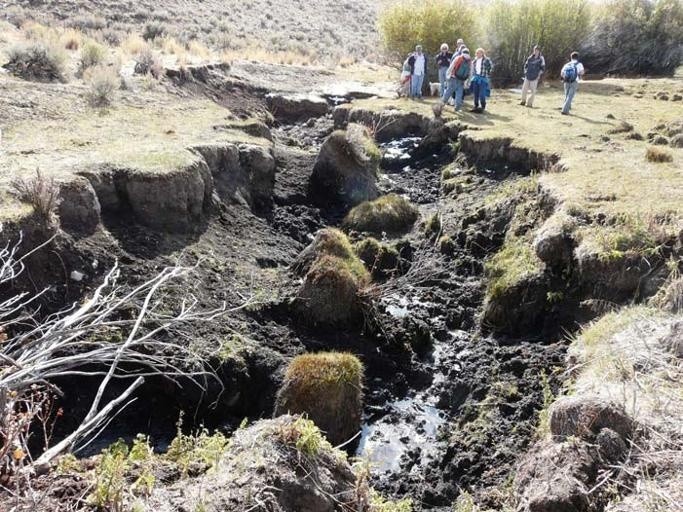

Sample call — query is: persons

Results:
[559,51,584,114]
[517,45,545,107]
[398,38,492,113]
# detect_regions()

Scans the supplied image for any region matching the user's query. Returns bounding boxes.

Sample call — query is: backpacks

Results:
[564,62,580,82]
[454,57,470,80]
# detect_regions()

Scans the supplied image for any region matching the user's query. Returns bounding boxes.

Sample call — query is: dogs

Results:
[429,81,447,96]
